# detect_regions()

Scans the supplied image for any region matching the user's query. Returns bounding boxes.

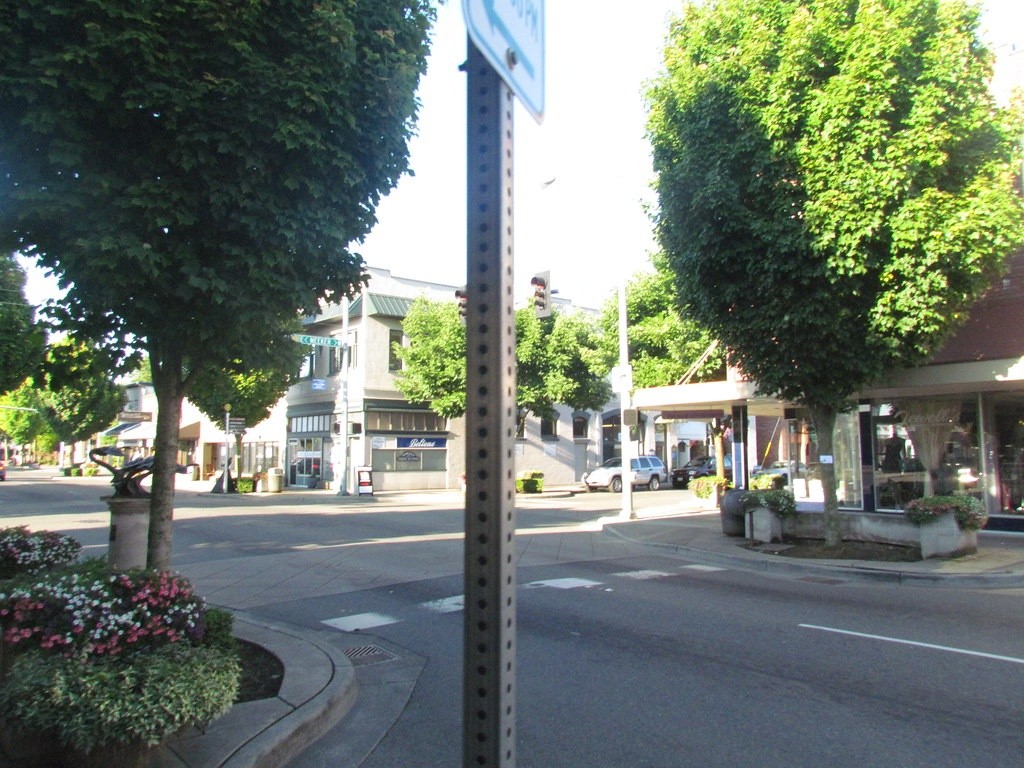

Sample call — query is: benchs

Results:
[203,463,215,479]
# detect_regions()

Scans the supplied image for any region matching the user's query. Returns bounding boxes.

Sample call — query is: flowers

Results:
[253,471,269,481]
[709,415,732,440]
[739,490,798,517]
[904,493,988,532]
[687,475,734,499]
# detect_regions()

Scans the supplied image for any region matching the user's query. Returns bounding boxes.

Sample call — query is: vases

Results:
[254,479,269,492]
[742,502,782,543]
[919,509,977,559]
[688,484,722,509]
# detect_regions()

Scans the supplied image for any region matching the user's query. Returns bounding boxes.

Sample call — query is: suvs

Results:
[581,456,666,493]
[669,456,732,489]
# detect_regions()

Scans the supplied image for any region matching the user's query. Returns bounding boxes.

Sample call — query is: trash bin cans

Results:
[267,468,284,493]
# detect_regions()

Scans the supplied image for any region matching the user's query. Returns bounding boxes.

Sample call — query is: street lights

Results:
[542,173,635,520]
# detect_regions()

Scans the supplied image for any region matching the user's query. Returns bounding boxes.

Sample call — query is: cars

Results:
[753,461,809,479]
[0,462,6,481]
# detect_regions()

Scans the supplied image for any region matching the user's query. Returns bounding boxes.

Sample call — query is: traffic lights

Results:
[531,271,551,319]
[455,285,466,325]
